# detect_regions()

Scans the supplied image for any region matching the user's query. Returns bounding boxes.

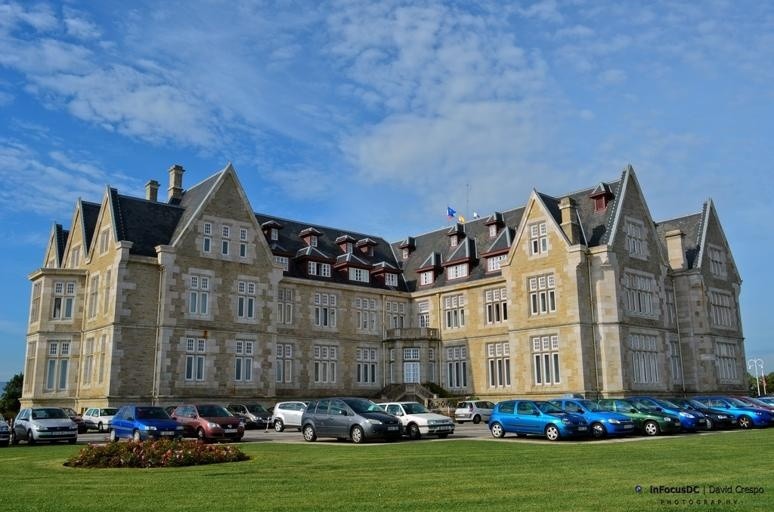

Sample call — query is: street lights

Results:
[747,358,765,397]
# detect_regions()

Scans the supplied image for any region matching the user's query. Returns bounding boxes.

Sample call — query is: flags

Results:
[447,207,455,220]
[457,215,466,224]
